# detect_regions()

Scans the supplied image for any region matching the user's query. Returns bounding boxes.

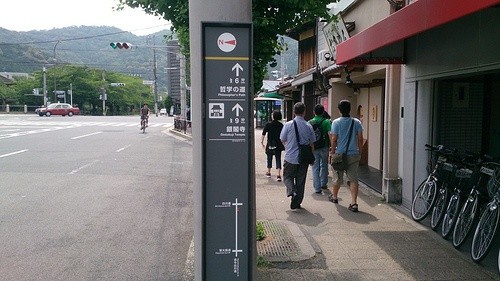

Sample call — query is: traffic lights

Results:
[110,41,132,50]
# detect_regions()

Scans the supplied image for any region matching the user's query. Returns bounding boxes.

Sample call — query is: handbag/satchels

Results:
[299,144,315,165]
[331,152,348,171]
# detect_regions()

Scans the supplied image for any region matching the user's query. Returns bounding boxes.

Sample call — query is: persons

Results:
[141,103,150,130]
[306,103,334,194]
[261,109,288,182]
[329,98,364,213]
[281,101,316,212]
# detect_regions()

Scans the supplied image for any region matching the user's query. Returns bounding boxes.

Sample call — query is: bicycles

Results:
[140,113,149,133]
[409,142,500,266]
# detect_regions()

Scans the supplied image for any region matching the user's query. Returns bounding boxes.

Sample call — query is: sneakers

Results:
[264,172,282,181]
[348,203,358,212]
[327,194,338,203]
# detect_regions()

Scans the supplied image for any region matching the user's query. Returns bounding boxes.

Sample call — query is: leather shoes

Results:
[286,191,305,212]
[315,185,328,193]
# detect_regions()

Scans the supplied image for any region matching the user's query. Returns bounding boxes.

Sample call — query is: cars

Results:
[35,102,79,117]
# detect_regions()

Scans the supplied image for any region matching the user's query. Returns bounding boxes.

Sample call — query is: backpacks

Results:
[310,118,326,150]
